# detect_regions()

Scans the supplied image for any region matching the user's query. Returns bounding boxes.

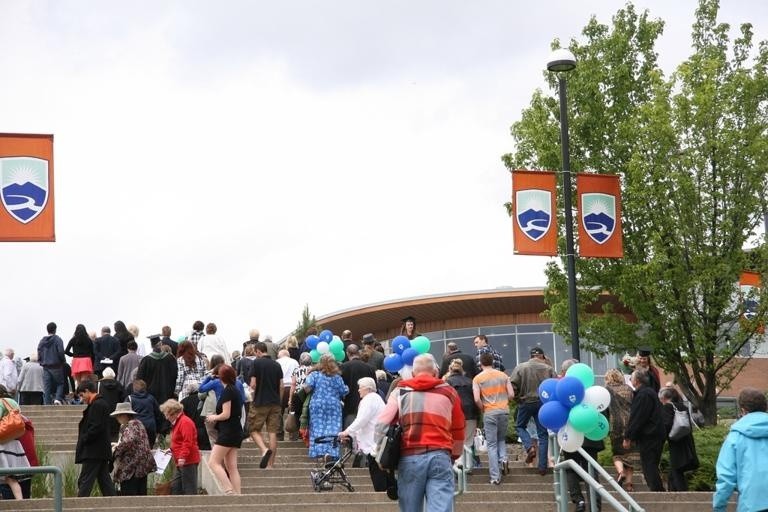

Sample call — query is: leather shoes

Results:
[575,503,586,511]
[525,446,536,463]
[539,470,547,476]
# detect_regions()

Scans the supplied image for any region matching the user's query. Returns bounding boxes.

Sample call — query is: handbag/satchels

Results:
[285,412,300,432]
[473,427,488,456]
[0,398,26,445]
[243,383,252,403]
[668,409,692,441]
[375,425,402,470]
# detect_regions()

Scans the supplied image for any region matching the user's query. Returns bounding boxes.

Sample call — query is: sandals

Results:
[618,471,627,485]
[625,483,635,492]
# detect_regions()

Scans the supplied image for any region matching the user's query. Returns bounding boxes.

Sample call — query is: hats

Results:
[638,348,651,357]
[401,317,417,322]
[24,357,30,362]
[532,347,546,360]
[146,334,167,345]
[110,402,139,416]
[361,333,377,343]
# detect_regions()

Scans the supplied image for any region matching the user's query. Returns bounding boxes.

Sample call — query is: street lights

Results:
[546,51,605,511]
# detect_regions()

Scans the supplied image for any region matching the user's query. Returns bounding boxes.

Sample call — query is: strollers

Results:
[309,435,356,492]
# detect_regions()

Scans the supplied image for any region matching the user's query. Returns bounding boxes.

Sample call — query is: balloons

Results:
[383,335,430,372]
[538,363,610,452]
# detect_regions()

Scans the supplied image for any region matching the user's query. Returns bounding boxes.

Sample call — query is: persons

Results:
[1,316,247,500]
[400,316,422,340]
[372,352,467,512]
[712,388,767,512]
[606,347,701,494]
[232,317,385,471]
[439,334,578,486]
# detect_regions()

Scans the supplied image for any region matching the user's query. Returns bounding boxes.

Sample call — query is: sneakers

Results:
[54,400,62,405]
[260,449,272,469]
[487,479,500,484]
[502,460,509,475]
